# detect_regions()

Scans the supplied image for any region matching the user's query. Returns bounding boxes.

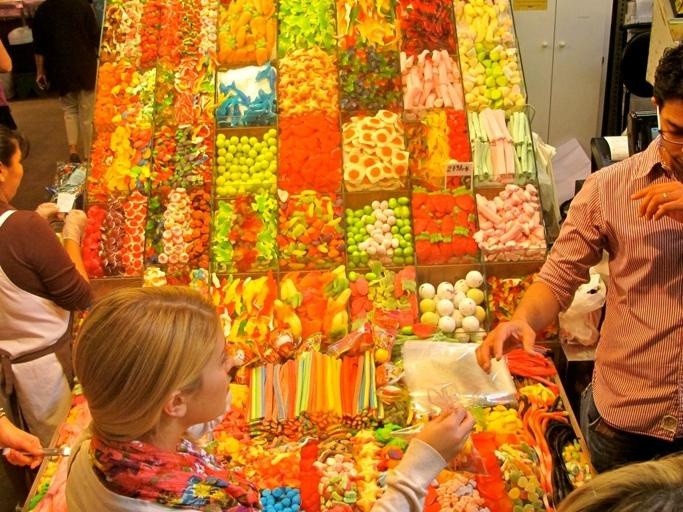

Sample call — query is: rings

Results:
[662,193,668,203]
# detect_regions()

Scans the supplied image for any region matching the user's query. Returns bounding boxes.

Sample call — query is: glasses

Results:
[656,104,683,146]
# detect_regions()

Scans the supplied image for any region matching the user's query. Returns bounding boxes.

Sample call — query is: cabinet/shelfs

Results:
[512,0,607,180]
[591,0,682,171]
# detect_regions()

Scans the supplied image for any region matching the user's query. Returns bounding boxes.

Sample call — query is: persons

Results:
[0,40,17,130]
[0,401,46,512]
[476,43,682,472]
[64,285,476,512]
[549,454,681,511]
[0,123,93,511]
[30,1,100,163]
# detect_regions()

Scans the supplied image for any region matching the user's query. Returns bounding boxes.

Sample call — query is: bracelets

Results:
[0,407,3,411]
[0,412,5,417]
